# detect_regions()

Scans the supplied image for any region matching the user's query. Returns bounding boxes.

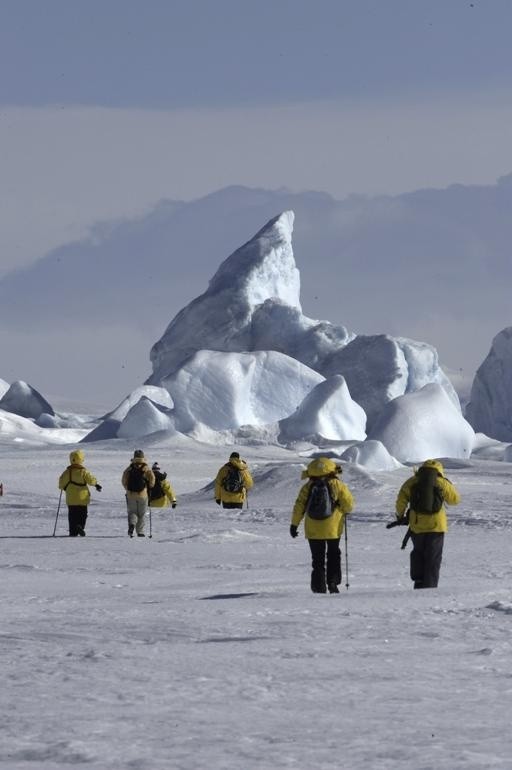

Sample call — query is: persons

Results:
[215,451,254,510]
[290,457,353,593]
[150,462,176,509]
[59,449,101,537]
[395,459,460,588]
[122,450,155,537]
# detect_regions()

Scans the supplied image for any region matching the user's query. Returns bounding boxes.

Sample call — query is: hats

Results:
[152,462,163,471]
[134,450,144,458]
[230,452,240,460]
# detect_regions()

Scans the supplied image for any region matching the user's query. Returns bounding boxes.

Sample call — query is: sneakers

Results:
[128,526,134,535]
[328,582,339,593]
[75,524,85,536]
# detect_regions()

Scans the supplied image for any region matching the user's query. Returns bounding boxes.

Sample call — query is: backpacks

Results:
[305,474,338,520]
[410,466,443,515]
[224,463,244,493]
[128,464,147,492]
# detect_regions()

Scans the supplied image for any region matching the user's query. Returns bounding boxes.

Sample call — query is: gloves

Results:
[290,525,299,537]
